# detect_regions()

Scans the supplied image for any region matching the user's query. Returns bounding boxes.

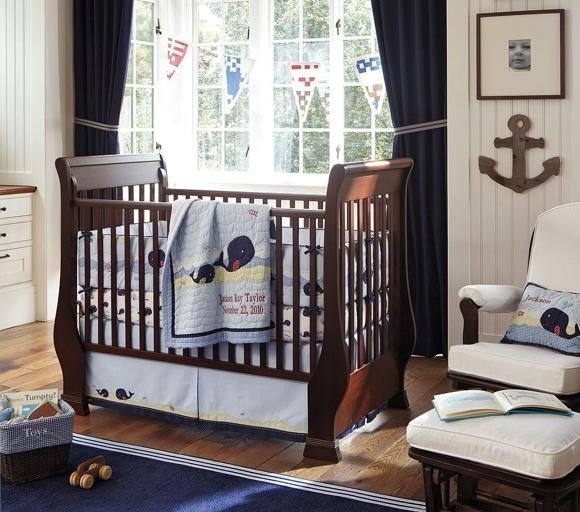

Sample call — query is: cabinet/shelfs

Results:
[0,184,37,332]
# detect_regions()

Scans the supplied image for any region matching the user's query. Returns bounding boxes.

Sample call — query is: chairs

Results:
[444,202,580,402]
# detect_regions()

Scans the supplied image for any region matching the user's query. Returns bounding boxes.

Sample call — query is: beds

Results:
[52,152,415,463]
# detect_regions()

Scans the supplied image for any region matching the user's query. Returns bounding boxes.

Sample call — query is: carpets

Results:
[1,430,427,512]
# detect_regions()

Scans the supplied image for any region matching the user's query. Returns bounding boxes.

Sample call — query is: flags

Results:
[353,56,386,114]
[289,61,321,122]
[164,37,191,79]
[223,54,256,113]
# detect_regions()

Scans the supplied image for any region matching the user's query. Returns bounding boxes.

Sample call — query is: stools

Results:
[407,392,580,511]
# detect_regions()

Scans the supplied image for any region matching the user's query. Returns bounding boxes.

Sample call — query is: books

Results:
[429,389,575,421]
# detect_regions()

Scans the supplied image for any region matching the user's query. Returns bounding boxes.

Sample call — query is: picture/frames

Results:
[474,7,567,101]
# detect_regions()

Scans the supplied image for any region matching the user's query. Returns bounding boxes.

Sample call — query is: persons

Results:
[508,40,531,71]
